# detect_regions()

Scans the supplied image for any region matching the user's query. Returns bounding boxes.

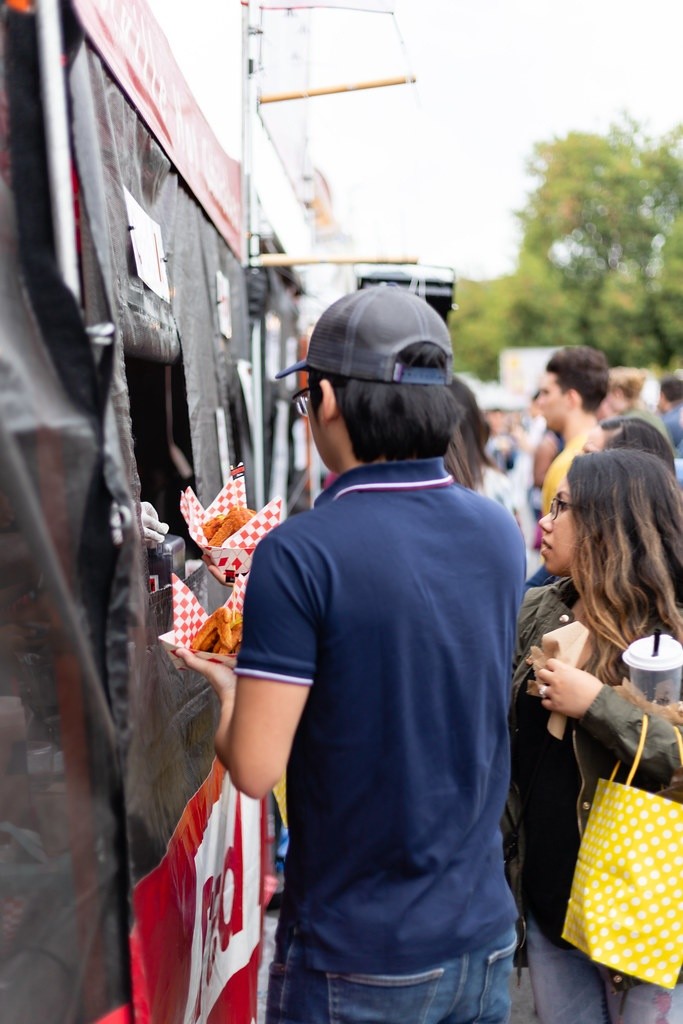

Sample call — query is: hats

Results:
[275,285,453,385]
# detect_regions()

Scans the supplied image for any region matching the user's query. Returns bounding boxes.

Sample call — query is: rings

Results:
[538,686,547,699]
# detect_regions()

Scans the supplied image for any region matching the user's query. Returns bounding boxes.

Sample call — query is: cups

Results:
[621,635,683,706]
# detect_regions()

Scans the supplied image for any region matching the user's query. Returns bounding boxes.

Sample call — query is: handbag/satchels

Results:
[560,714,683,990]
[501,813,519,862]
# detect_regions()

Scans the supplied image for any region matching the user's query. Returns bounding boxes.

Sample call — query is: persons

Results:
[436,345,683,1024]
[140,501,169,549]
[173,284,522,1023]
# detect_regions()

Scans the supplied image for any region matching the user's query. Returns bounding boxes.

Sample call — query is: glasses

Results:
[292,387,311,417]
[550,498,599,520]
